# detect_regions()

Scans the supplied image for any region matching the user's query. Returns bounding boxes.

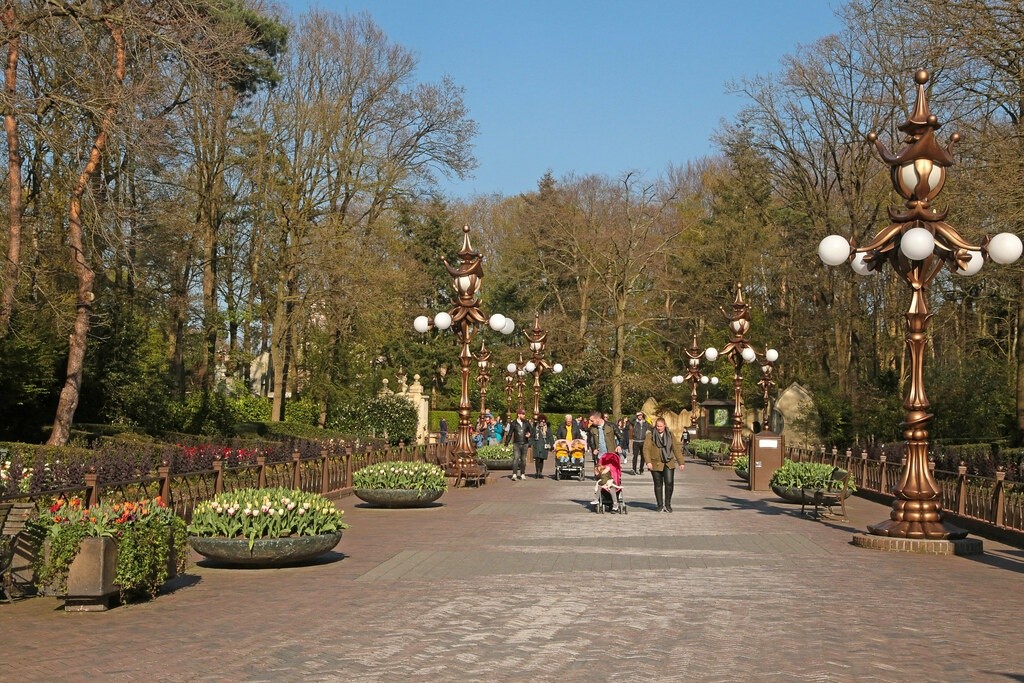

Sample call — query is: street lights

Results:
[705,281,778,465]
[468,313,515,430]
[503,352,527,443]
[818,70,1023,540]
[523,314,563,428]
[413,224,507,477]
[672,334,718,428]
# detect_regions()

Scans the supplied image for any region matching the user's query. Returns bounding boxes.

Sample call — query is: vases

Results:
[352,487,444,506]
[189,529,342,565]
[483,458,527,470]
[44,536,185,612]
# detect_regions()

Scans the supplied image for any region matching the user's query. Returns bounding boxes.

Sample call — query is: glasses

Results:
[604,417,607,418]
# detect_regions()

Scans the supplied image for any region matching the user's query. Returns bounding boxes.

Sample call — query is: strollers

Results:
[552,439,587,481]
[594,450,628,514]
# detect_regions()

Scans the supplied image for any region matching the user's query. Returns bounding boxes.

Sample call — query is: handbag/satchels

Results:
[544,443,552,449]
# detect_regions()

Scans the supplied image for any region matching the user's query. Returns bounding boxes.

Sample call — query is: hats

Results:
[507,416,511,419]
[517,409,525,414]
[636,412,643,416]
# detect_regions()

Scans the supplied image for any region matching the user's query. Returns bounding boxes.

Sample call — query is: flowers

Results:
[474,441,516,459]
[186,484,352,551]
[22,495,192,611]
[351,460,450,499]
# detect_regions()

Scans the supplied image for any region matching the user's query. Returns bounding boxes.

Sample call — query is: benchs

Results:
[801,466,851,522]
[455,458,489,489]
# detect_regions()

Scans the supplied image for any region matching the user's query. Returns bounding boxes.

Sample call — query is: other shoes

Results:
[681,452,685,454]
[560,462,567,466]
[569,463,575,467]
[535,474,543,478]
[578,463,583,466]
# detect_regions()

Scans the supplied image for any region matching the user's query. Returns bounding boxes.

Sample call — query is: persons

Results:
[642,417,685,513]
[439,408,691,481]
[590,463,619,511]
[588,410,626,505]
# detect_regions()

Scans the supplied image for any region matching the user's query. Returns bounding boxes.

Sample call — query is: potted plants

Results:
[768,457,859,504]
[733,454,749,481]
[687,438,730,462]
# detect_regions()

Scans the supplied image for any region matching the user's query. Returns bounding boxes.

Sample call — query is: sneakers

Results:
[511,474,517,481]
[521,474,526,480]
[587,450,589,453]
[656,506,672,513]
[623,458,627,464]
[639,469,644,474]
[612,505,618,511]
[590,499,599,504]
[630,469,636,475]
